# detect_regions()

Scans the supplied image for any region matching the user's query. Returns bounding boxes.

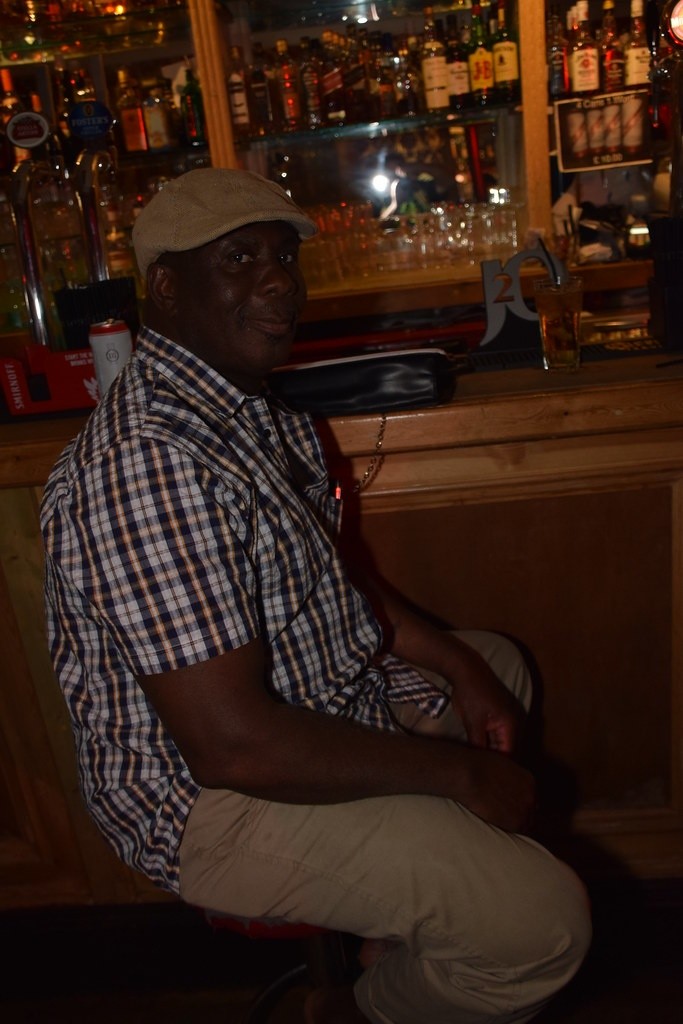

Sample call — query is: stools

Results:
[192,905,359,1024]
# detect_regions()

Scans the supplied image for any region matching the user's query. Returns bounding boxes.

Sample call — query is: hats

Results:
[132,167,316,279]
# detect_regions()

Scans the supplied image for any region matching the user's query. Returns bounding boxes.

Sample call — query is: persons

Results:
[40,168,592,1024]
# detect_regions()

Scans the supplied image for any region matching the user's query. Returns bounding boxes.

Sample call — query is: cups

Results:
[527,275,597,374]
[299,201,518,286]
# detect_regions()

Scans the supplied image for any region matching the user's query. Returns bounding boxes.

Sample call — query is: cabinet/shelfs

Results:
[0,0,681,357]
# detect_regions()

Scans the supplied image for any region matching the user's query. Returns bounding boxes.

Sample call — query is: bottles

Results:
[545,0,654,99]
[140,78,171,148]
[31,93,53,160]
[421,1,521,116]
[565,95,645,167]
[180,69,208,150]
[92,137,139,280]
[0,69,32,163]
[393,40,422,120]
[27,149,94,353]
[226,31,382,138]
[116,69,149,153]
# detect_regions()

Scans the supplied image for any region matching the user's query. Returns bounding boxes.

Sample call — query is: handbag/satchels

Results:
[273,347,455,417]
